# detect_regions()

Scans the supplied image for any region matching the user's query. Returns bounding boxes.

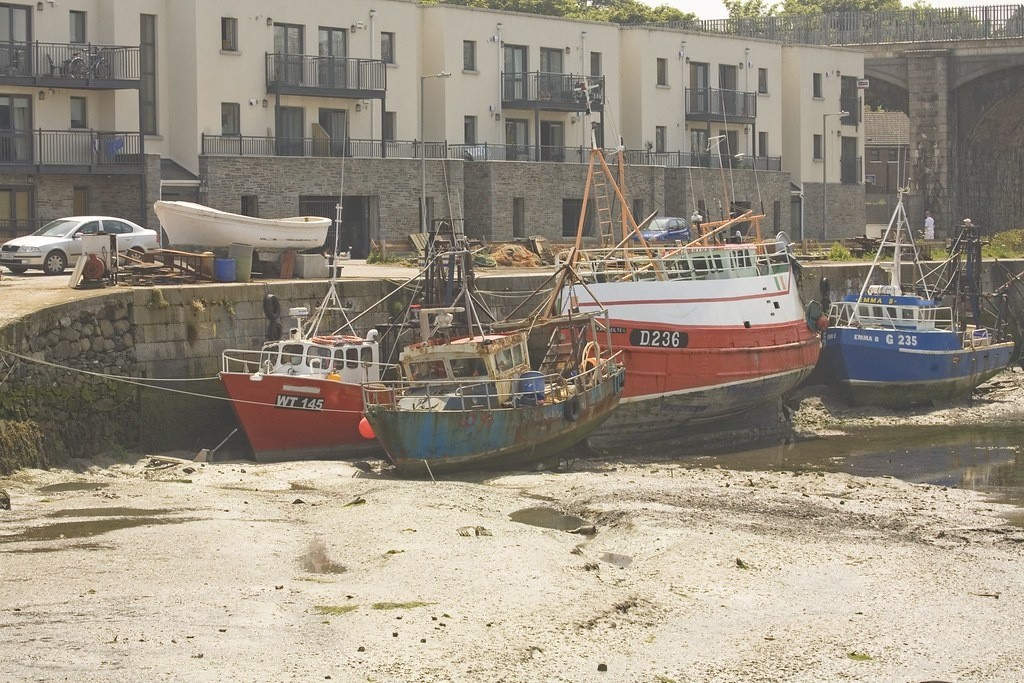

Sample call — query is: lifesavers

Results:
[309,334,365,347]
[564,393,581,422]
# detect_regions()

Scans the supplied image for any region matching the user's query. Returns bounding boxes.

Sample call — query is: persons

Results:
[963,218,982,244]
[924,212,935,251]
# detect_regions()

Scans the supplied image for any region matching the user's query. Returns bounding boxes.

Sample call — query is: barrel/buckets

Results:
[215,258,237,281]
[519,370,546,400]
[229,242,253,282]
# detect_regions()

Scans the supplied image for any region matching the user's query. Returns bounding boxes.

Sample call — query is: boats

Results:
[217,102,627,477]
[153,198,333,254]
[547,127,824,453]
[816,128,1017,408]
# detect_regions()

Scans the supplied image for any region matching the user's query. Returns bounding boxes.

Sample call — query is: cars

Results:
[1,214,159,276]
[632,217,689,244]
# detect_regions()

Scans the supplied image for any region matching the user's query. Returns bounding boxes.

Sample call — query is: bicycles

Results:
[66,47,110,79]
